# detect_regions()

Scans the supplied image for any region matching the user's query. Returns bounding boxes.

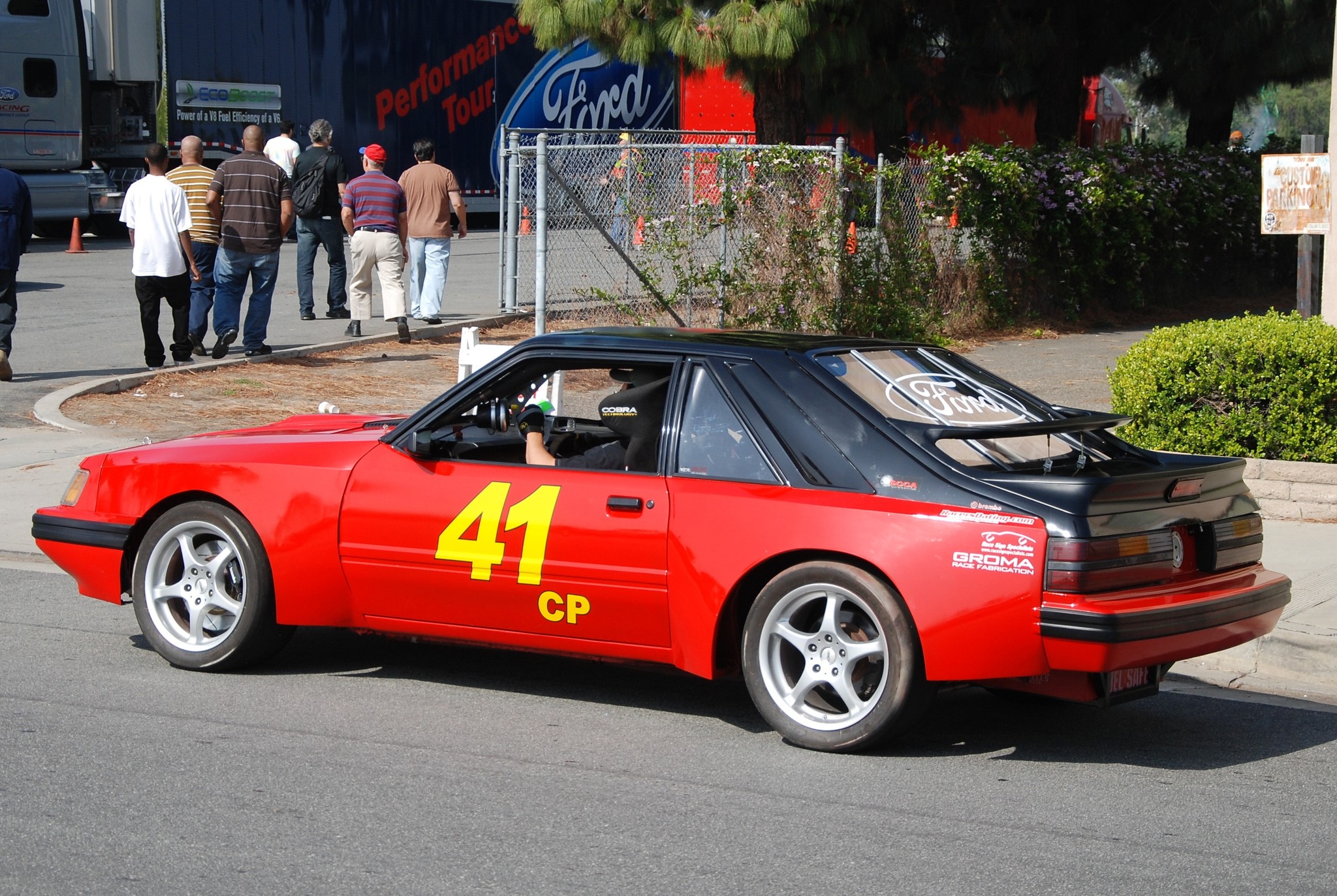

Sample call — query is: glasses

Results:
[361,157,367,161]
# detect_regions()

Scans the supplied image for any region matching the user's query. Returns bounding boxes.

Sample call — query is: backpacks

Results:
[291,147,332,218]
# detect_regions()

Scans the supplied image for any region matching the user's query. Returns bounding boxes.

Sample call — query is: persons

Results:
[0,167,33,381]
[206,125,293,359]
[293,120,350,320]
[516,367,664,470]
[163,135,225,354]
[400,140,468,324]
[340,145,410,344]
[265,121,301,242]
[119,145,202,371]
[599,133,644,252]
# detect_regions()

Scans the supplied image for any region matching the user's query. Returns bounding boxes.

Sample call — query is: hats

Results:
[608,363,673,387]
[359,144,386,164]
[620,133,634,142]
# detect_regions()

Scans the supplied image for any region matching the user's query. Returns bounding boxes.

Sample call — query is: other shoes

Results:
[326,305,350,318]
[244,343,272,356]
[188,330,207,355]
[172,355,194,366]
[603,245,615,251]
[0,350,13,381]
[212,328,237,359]
[423,317,443,324]
[146,364,165,370]
[301,311,315,319]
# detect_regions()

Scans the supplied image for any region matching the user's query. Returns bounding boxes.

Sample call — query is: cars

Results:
[29,331,1293,751]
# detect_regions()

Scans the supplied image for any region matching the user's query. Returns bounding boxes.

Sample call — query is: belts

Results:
[355,227,384,232]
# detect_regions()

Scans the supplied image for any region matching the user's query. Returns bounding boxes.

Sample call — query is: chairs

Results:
[598,377,671,472]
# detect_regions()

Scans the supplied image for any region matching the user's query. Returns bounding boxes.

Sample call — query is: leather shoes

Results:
[344,321,361,336]
[397,319,411,344]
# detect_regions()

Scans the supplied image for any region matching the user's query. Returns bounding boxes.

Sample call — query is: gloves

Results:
[516,404,545,439]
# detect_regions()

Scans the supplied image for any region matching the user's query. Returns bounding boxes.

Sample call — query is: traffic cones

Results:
[632,217,649,248]
[844,222,858,253]
[520,205,536,236]
[945,207,957,229]
[65,218,89,254]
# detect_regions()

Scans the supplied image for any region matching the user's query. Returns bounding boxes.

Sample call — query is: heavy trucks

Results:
[3,0,682,241]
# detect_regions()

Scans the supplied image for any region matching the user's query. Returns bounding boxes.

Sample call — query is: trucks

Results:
[678,24,1152,230]
